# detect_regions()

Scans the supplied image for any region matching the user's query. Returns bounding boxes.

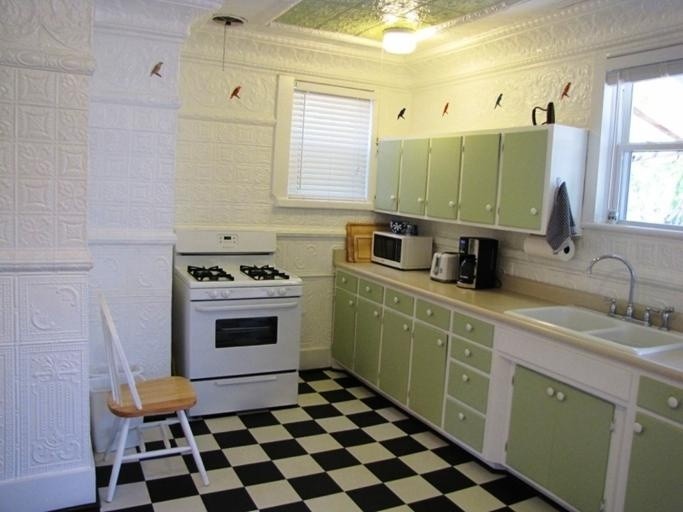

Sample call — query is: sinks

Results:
[502,304,627,335]
[582,324,683,355]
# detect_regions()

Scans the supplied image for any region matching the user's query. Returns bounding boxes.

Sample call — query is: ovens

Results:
[183,296,303,417]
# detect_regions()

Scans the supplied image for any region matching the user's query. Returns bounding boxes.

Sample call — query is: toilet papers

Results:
[523,235,576,262]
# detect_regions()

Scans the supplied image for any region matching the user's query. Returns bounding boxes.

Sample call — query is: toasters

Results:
[429,251,460,283]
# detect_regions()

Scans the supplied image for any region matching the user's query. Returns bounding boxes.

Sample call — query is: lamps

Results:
[382,25,419,55]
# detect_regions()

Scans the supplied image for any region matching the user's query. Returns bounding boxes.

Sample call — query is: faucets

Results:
[585,254,636,318]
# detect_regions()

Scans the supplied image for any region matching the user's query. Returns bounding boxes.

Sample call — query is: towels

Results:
[546,180,584,253]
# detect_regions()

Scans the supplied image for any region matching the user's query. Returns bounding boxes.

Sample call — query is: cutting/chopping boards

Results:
[344,222,393,263]
[352,234,373,264]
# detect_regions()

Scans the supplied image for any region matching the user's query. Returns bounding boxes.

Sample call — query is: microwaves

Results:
[370,230,435,272]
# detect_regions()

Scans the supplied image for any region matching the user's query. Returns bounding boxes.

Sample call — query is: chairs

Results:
[94,291,212,505]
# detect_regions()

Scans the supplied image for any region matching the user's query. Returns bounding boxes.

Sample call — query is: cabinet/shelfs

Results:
[410,290,451,429]
[498,124,589,238]
[443,300,494,455]
[374,137,400,219]
[503,363,613,509]
[353,276,382,393]
[378,284,414,407]
[621,370,683,512]
[459,129,501,228]
[425,131,462,223]
[397,135,428,222]
[330,267,358,379]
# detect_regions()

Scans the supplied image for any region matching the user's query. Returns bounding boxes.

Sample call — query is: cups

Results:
[390,220,417,236]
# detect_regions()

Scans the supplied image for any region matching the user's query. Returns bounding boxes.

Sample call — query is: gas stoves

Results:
[172,254,304,289]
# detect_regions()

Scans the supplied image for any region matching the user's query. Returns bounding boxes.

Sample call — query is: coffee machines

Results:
[456,236,499,291]
